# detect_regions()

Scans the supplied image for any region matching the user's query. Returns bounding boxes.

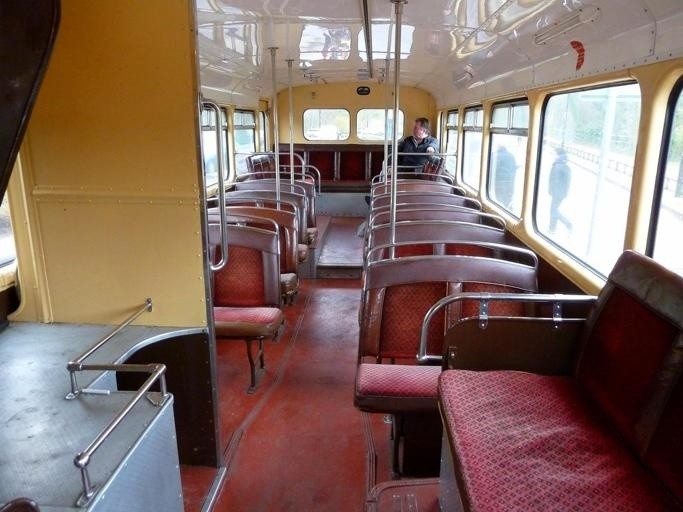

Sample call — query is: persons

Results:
[493,143,518,207]
[362,116,439,206]
[542,146,573,233]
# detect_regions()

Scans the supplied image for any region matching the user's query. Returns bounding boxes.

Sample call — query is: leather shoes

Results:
[365,196,370,205]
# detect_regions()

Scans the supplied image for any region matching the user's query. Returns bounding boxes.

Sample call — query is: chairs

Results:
[204,144,338,396]
